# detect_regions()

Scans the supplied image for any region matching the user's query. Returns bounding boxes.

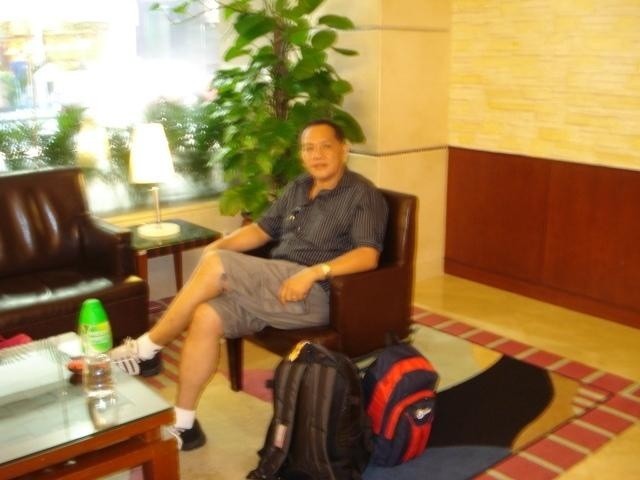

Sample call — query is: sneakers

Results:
[105,343,161,377]
[168,419,206,450]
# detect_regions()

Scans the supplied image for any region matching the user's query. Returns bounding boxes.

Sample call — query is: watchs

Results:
[321,264,333,281]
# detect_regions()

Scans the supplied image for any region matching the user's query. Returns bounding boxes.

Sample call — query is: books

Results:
[0,357,71,404]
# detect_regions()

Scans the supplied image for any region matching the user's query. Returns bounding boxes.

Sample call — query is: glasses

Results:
[286,204,309,225]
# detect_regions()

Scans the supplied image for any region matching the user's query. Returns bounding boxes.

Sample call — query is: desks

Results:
[128,219,223,293]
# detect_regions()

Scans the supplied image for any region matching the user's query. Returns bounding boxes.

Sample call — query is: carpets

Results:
[96,294,640,480]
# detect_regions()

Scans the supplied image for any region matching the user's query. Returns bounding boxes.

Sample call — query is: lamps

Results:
[131,124,180,238]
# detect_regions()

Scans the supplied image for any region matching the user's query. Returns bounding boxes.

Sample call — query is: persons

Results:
[103,116,393,451]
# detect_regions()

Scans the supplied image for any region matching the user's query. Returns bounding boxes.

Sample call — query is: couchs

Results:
[0,166,149,348]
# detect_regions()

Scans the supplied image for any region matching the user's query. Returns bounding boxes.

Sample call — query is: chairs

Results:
[225,191,419,393]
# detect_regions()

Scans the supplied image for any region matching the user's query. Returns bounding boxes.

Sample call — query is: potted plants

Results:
[147,0,366,228]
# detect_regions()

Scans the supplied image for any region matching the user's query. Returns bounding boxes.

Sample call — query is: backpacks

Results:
[246,331,438,480]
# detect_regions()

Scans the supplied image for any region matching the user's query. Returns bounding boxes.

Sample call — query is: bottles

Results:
[79,301,117,401]
